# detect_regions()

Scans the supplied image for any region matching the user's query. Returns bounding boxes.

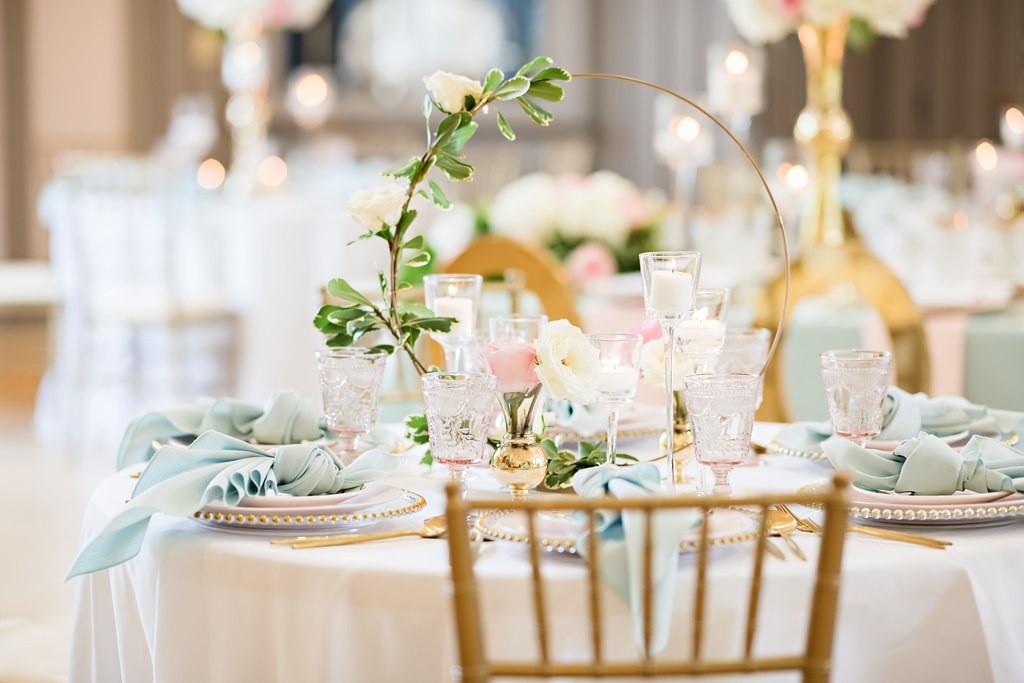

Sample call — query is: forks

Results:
[804,517,946,550]
[774,503,953,547]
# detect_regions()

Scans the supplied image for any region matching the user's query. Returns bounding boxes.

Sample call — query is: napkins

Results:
[66,387,1024,658]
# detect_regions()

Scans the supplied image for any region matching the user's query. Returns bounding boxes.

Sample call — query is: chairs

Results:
[424,235,573,372]
[446,468,850,683]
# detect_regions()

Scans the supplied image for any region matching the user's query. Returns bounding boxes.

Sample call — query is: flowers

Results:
[173,0,327,35]
[727,1,934,45]
[311,54,568,359]
[493,170,662,278]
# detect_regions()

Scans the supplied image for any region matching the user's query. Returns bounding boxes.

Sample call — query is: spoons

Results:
[291,514,448,549]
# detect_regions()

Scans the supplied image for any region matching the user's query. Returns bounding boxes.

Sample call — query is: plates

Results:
[191,485,426,528]
[473,507,759,553]
[797,480,1023,524]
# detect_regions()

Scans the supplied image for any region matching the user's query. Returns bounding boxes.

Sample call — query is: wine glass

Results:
[318,253,891,535]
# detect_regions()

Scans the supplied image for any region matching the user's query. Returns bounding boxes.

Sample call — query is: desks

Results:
[47,419,1024,683]
